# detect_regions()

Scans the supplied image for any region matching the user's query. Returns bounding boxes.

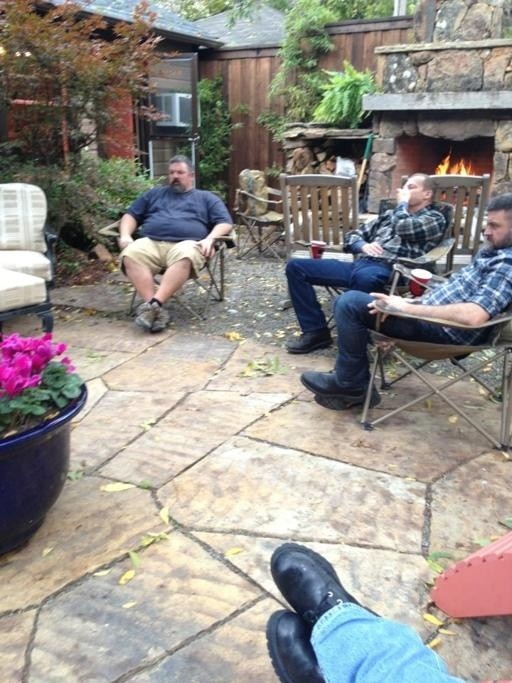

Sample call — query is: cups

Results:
[410,269,433,295]
[311,240,326,258]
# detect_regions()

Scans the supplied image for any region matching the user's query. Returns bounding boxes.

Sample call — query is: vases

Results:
[0,370,90,567]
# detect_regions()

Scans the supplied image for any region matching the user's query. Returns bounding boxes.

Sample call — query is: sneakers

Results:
[136,301,170,333]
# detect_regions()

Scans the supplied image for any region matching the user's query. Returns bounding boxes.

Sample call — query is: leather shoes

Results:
[301,371,380,409]
[287,331,333,354]
[270,543,381,624]
[266,609,325,683]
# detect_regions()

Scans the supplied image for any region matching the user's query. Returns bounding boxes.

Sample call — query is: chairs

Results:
[360,293,511,462]
[320,198,456,347]
[400,173,491,273]
[281,173,360,309]
[235,167,286,262]
[98,218,239,321]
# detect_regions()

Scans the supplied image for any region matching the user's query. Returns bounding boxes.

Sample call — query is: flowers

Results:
[0,333,89,436]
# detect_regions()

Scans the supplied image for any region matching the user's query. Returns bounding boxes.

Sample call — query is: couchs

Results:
[0,181,63,288]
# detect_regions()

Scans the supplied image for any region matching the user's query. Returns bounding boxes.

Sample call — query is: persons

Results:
[287,173,446,354]
[117,155,234,333]
[300,197,512,412]
[266,545,466,683]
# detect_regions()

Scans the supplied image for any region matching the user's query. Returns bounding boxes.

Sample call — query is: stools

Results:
[0,265,56,335]
